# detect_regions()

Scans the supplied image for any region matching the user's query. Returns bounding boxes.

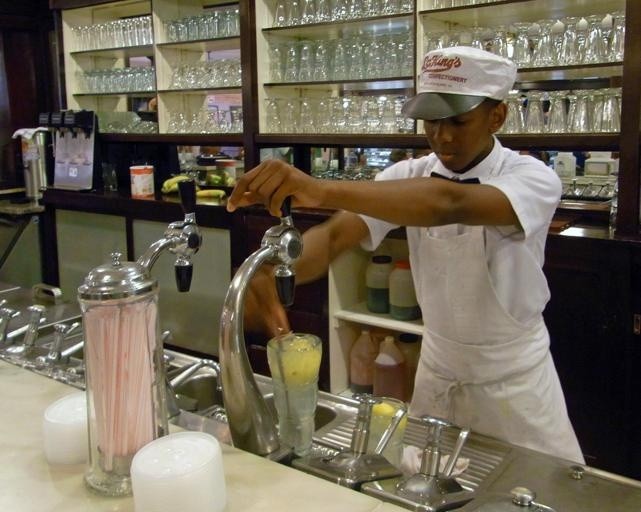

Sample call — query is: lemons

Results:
[372,401,394,423]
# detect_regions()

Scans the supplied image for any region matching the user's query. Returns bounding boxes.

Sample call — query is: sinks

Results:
[176,372,261,421]
[44,330,84,363]
[255,391,337,444]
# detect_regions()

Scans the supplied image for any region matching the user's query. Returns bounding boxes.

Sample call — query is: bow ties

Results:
[430,172,482,185]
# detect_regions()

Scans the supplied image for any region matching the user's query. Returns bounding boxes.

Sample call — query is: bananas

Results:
[161,176,200,193]
[196,190,226,200]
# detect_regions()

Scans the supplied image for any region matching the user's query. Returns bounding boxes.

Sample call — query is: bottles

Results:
[554,152,576,177]
[217,158,237,182]
[129,165,155,198]
[585,151,615,176]
[367,255,396,313]
[389,260,421,320]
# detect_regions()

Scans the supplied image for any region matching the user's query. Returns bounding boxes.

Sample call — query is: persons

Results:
[222,46,585,464]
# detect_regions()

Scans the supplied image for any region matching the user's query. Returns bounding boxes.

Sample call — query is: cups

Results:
[267,1,412,132]
[369,397,406,470]
[526,92,543,133]
[571,89,597,133]
[609,11,627,61]
[512,21,532,66]
[424,30,444,52]
[73,19,156,130]
[534,18,556,68]
[582,13,606,62]
[446,28,461,49]
[599,86,621,133]
[264,333,322,451]
[559,15,579,66]
[545,90,568,132]
[469,25,487,49]
[505,91,521,133]
[163,9,243,133]
[490,24,508,58]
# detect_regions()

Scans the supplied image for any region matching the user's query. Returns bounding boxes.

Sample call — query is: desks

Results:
[1,280,641,510]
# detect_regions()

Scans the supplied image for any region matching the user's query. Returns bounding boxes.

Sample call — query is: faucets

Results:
[46,320,84,367]
[163,358,222,416]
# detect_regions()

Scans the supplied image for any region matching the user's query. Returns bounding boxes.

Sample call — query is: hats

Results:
[401,46,516,121]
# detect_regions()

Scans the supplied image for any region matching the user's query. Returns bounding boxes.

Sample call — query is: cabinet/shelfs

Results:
[240,202,640,486]
[41,187,240,367]
[45,0,641,230]
